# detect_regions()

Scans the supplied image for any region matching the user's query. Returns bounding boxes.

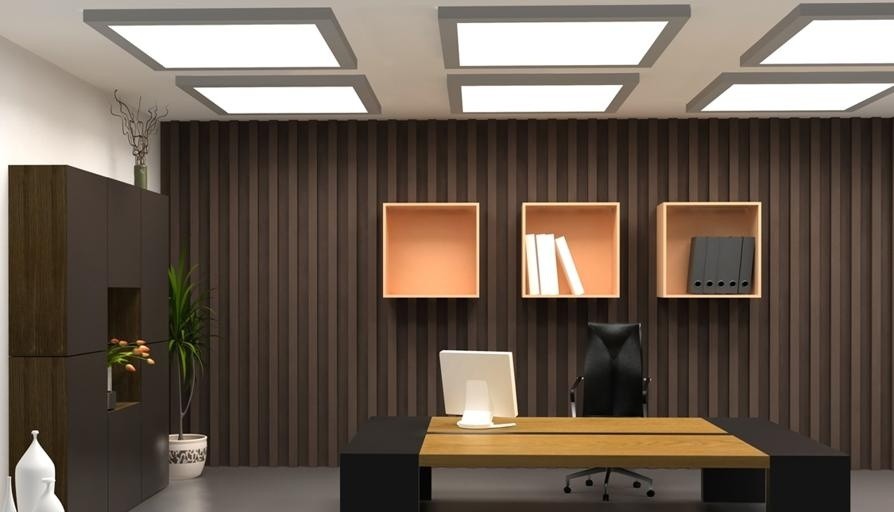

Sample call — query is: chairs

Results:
[562,319,655,503]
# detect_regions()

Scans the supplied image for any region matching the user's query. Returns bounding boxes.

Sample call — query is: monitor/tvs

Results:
[440,348,519,427]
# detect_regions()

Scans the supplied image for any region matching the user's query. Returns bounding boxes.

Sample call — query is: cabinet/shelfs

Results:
[378,197,767,302]
[5,164,168,358]
[8,340,171,511]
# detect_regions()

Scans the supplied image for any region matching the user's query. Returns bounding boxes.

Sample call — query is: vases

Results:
[13,429,57,512]
[30,477,65,512]
[133,155,147,190]
[0,475,17,512]
[105,363,117,412]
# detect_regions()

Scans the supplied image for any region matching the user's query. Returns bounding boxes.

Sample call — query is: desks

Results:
[337,410,852,511]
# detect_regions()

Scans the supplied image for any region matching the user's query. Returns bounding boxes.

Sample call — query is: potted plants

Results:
[165,244,224,481]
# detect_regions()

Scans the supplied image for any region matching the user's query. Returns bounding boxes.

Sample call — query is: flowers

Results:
[105,336,156,374]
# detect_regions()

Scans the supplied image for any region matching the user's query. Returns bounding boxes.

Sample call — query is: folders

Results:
[687,236,755,294]
